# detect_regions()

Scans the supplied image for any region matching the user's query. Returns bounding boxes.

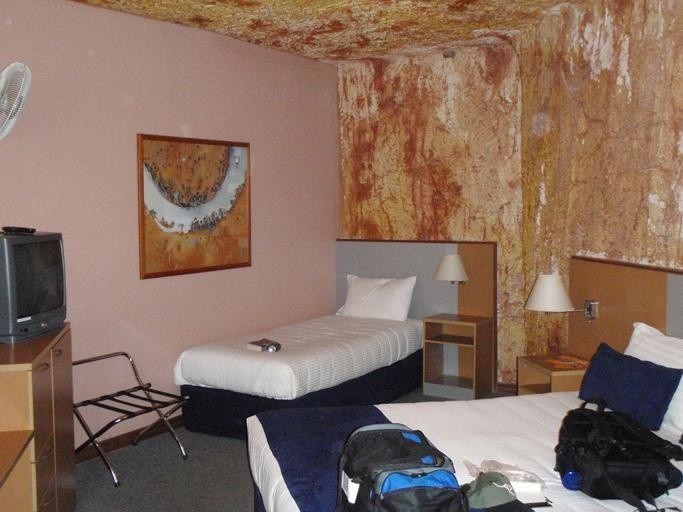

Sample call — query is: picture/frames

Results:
[137,134,252,282]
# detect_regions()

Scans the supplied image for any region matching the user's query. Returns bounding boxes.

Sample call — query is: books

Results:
[244,338,277,352]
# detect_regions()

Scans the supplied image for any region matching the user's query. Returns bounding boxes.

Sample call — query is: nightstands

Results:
[515,353,589,395]
[423,313,493,400]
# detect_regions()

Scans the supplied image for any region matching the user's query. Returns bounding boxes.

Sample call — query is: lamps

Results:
[435,254,470,286]
[523,272,600,319]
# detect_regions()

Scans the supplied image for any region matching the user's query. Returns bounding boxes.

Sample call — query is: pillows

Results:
[621,322,683,438]
[336,274,416,321]
[577,342,682,431]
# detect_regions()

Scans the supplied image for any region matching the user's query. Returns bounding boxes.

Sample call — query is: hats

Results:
[467,471,535,512]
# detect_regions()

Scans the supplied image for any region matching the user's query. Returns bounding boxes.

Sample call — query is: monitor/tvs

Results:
[0,233,66,345]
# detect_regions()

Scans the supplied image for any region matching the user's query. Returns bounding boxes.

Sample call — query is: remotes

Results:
[3,227,36,234]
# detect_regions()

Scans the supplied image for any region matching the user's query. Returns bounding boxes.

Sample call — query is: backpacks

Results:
[555,398,683,500]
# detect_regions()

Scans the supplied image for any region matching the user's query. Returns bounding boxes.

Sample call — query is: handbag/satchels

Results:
[337,423,466,511]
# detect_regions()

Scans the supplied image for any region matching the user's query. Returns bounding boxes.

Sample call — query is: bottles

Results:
[559,458,581,491]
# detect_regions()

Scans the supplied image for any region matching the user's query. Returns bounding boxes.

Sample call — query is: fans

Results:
[1,60,33,140]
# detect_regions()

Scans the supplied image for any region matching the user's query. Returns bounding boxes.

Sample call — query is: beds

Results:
[245,391,683,512]
[173,317,424,442]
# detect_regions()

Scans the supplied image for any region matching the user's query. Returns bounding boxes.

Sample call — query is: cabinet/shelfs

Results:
[0,321,79,512]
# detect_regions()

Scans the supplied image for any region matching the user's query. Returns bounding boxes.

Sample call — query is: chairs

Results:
[65,351,190,487]
